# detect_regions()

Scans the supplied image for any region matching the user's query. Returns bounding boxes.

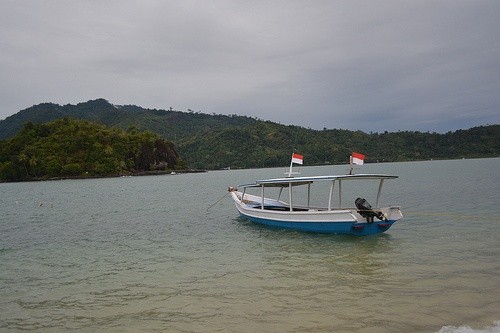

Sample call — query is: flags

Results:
[352,151,365,166]
[291,153,304,165]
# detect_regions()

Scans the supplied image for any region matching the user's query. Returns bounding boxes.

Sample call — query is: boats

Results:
[225,152,403,239]
[283,172,302,175]
[170,172,177,175]
